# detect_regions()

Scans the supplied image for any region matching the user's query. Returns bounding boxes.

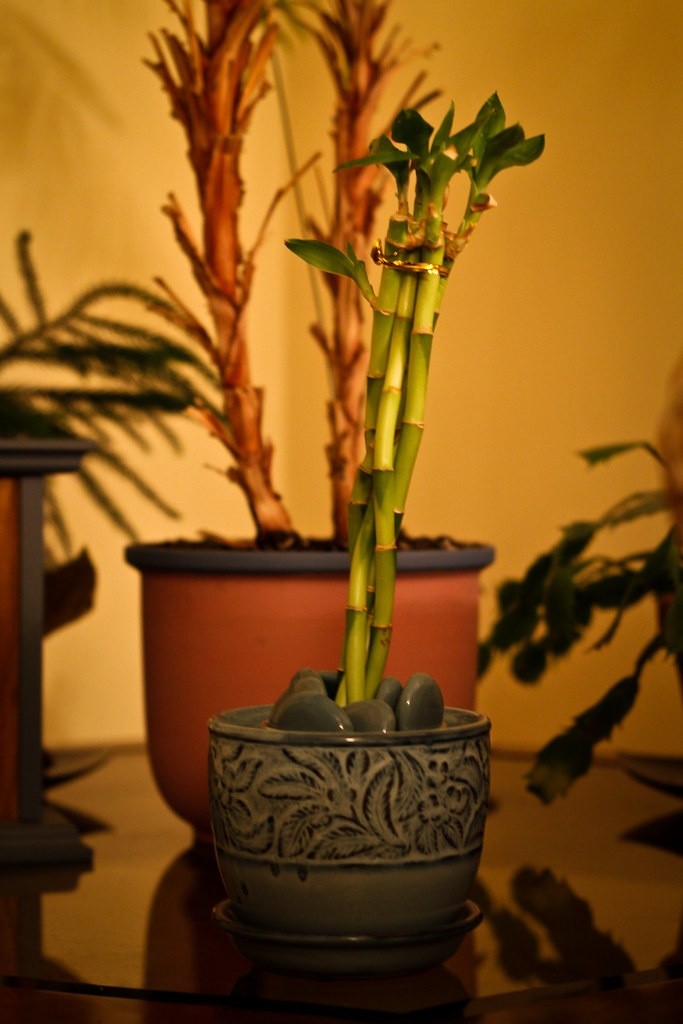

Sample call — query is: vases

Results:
[120,0,496,829]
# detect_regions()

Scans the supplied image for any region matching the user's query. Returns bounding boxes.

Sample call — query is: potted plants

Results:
[206,96,545,981]
